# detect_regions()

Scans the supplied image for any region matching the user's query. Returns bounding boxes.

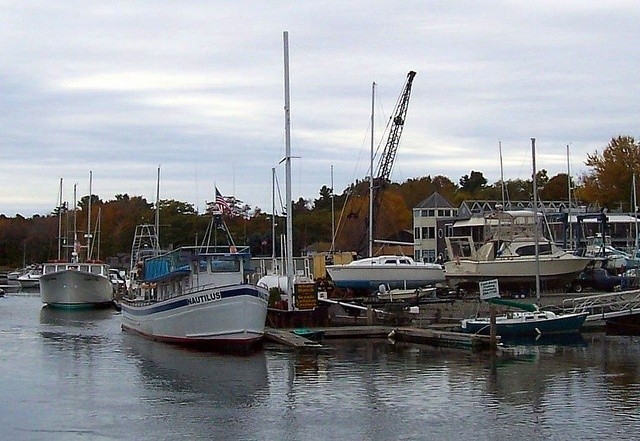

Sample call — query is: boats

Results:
[325,255,446,289]
[18,268,39,288]
[8,272,17,280]
[464,309,590,346]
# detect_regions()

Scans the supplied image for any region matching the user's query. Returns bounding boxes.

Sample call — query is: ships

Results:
[445,211,593,289]
[39,263,113,306]
[120,246,268,348]
